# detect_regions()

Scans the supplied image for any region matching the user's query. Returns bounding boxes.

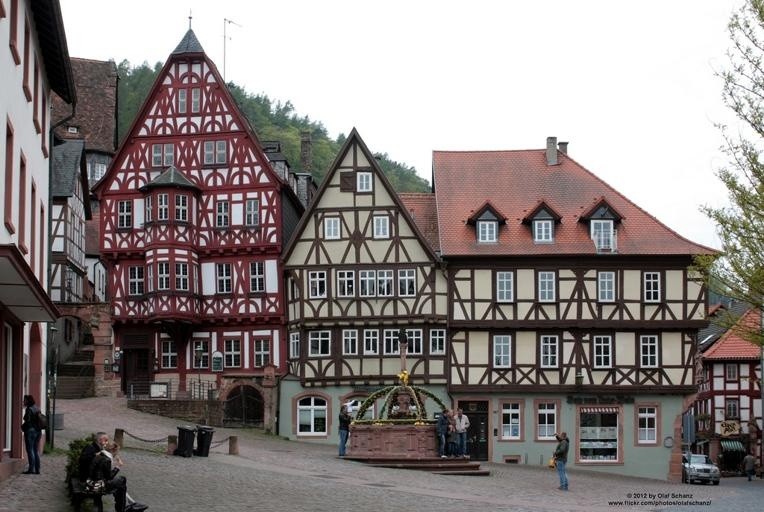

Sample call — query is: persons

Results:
[339,405,352,456]
[553,432,569,490]
[79,432,149,512]
[743,452,754,481]
[21,395,42,474]
[437,408,470,458]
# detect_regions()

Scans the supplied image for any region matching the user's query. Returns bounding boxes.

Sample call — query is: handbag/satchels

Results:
[85,478,107,495]
[548,457,556,468]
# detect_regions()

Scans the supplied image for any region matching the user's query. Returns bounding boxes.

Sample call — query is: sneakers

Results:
[23,468,41,474]
[125,503,149,512]
[439,454,471,460]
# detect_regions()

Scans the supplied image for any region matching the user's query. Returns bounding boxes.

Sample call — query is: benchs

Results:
[66,462,103,511]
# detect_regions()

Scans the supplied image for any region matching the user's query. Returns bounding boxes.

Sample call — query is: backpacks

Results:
[26,406,49,430]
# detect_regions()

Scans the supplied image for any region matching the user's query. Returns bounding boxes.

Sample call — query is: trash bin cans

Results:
[177,424,216,457]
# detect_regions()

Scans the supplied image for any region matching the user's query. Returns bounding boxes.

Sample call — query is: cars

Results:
[682,453,721,484]
